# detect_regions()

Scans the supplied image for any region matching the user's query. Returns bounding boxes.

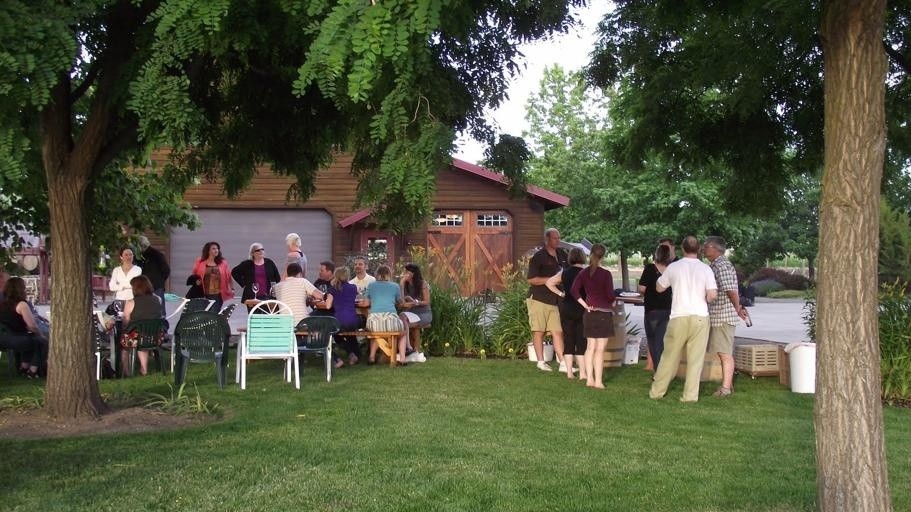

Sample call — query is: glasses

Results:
[252,248,264,252]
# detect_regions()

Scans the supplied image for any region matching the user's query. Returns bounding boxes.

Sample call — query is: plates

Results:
[619,292,641,298]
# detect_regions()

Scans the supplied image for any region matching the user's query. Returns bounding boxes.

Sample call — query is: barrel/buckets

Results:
[784,342,816,393]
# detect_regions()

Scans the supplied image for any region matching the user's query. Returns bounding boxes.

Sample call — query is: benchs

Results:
[265,300,419,368]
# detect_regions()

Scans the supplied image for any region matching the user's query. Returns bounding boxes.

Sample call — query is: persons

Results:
[93,235,170,379]
[192,233,433,369]
[638,235,749,404]
[0,271,50,380]
[526,228,617,390]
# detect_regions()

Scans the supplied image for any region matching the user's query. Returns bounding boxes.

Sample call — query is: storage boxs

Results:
[527,342,555,363]
[778,344,790,387]
[678,349,730,382]
[789,340,817,394]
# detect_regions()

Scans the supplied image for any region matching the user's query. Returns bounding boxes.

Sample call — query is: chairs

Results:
[235,299,300,390]
[293,316,340,382]
[93,298,169,380]
[175,310,231,390]
[1,304,49,380]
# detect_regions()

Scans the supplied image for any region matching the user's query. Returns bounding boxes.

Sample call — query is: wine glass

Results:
[251,282,260,300]
[357,285,365,296]
[320,284,327,303]
[113,300,124,320]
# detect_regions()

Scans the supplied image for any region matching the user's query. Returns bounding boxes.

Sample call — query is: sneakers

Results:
[558,360,580,373]
[368,358,377,365]
[536,361,553,372]
[333,360,344,369]
[18,367,42,379]
[393,351,426,369]
[347,355,359,366]
[712,385,734,397]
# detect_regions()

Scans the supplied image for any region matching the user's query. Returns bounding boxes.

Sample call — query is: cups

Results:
[270,281,277,287]
